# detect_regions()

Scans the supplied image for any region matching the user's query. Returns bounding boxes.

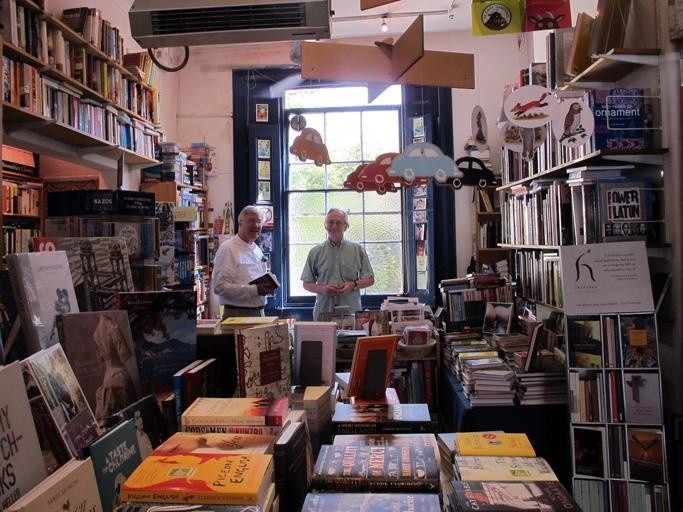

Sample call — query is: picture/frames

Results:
[251,100,274,270]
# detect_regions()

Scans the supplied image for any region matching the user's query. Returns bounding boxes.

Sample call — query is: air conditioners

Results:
[126,0,336,50]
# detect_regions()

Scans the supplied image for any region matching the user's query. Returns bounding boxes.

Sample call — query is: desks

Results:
[441,366,575,499]
[198,331,239,397]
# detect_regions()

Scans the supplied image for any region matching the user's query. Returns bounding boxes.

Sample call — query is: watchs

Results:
[353,280,358,292]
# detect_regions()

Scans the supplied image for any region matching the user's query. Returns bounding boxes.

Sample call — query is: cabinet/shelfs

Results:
[332,325,443,432]
[489,47,682,356]
[137,174,213,323]
[0,167,46,266]
[474,170,511,280]
[0,1,164,173]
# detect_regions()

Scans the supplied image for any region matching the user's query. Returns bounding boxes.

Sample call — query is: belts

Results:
[225,305,264,310]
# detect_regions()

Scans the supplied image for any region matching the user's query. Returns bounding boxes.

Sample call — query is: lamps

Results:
[379,10,389,33]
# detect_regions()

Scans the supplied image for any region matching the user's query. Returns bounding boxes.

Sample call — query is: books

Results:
[355,273,505,334]
[477,1,674,324]
[1,324,682,510]
[1,1,164,162]
[248,272,280,291]
[1,160,142,353]
[139,142,222,320]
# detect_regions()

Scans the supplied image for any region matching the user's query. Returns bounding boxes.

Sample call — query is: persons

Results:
[301,207,375,322]
[212,205,280,319]
